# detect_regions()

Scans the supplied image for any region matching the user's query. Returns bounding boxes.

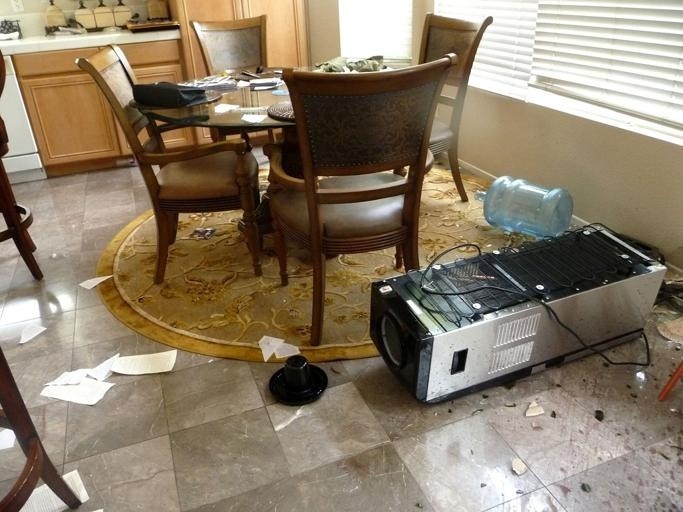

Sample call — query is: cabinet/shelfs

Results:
[173,0,307,147]
[11,36,195,178]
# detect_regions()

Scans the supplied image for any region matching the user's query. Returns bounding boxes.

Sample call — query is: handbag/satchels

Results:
[133,81,207,108]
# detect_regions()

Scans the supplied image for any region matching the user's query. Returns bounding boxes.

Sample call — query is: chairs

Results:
[188,13,275,157]
[0,344,83,511]
[77,43,262,285]
[360,13,493,204]
[263,52,458,345]
[0,53,42,285]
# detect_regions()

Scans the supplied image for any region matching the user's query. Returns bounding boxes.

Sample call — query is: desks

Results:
[137,66,402,188]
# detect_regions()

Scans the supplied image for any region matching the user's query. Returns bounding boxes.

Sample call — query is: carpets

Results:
[99,156,543,366]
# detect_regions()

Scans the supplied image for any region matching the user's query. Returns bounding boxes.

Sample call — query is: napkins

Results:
[241,113,269,123]
[214,103,239,113]
[237,77,284,92]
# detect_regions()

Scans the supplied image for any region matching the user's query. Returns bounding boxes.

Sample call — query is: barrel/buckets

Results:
[474,175,575,240]
